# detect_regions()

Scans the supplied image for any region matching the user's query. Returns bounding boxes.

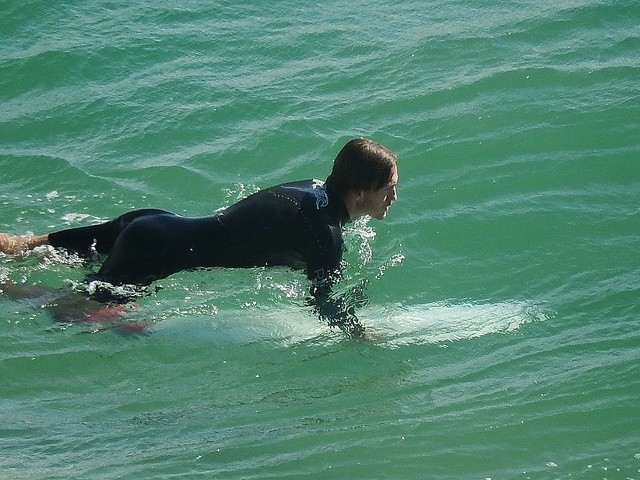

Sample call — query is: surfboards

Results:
[137,299,556,349]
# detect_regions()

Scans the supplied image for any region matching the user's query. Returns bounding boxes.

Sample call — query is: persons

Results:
[0,139,399,341]
